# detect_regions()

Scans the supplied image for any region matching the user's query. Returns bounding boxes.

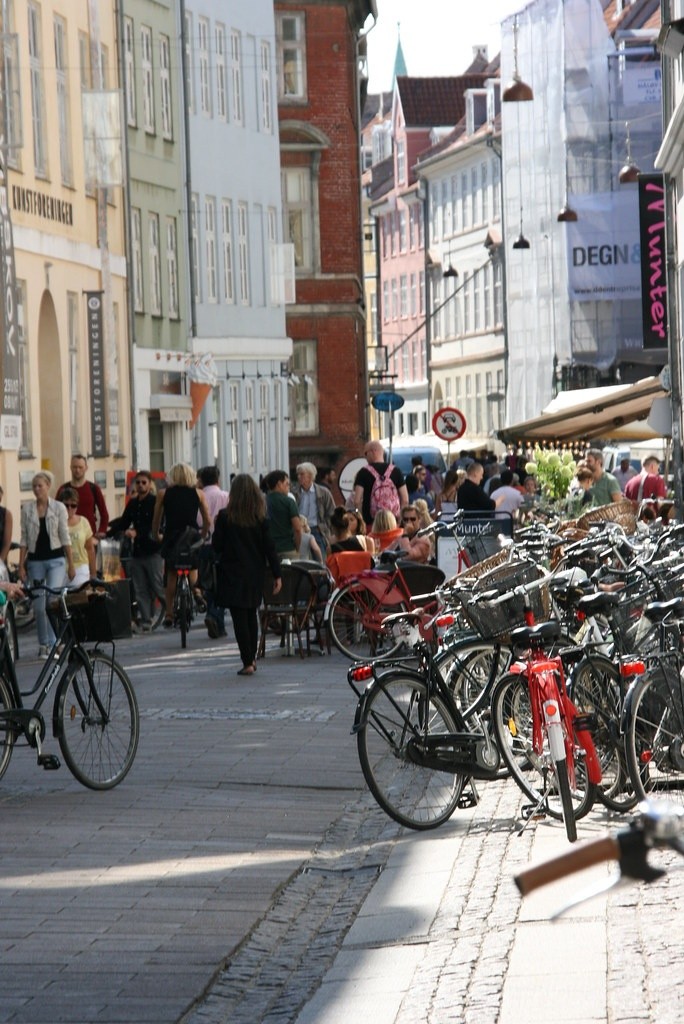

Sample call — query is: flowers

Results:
[525,445,577,502]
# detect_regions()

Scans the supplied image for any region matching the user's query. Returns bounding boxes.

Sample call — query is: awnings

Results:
[495,377,672,450]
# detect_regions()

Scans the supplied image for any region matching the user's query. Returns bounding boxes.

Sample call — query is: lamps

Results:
[618,121,642,185]
[443,239,458,277]
[558,0,578,222]
[513,101,531,249]
[502,14,535,102]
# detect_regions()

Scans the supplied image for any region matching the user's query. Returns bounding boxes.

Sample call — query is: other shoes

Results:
[38,647,59,659]
[309,638,332,646]
[237,666,254,675]
[139,620,152,631]
[252,661,257,670]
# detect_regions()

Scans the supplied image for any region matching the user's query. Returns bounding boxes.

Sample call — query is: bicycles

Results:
[169,526,212,649]
[323,508,684,845]
[1,578,140,792]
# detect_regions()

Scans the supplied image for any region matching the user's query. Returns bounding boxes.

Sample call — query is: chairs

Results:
[256,527,447,657]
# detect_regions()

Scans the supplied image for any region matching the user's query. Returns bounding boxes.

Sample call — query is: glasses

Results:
[64,503,77,509]
[136,480,147,485]
[365,451,368,455]
[403,517,416,522]
[419,472,426,476]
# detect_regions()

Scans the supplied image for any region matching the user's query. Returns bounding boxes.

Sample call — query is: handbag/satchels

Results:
[95,538,125,581]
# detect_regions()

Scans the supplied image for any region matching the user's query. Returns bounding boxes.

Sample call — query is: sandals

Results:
[194,592,207,612]
[162,614,173,627]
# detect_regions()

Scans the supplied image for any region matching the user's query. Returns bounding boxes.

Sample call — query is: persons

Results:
[568,450,638,517]
[0,488,27,606]
[212,473,282,675]
[260,460,374,646]
[116,463,230,638]
[353,441,541,562]
[18,470,76,660]
[54,456,110,554]
[625,456,667,504]
[60,489,97,587]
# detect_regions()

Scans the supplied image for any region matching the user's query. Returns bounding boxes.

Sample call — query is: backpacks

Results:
[363,464,400,519]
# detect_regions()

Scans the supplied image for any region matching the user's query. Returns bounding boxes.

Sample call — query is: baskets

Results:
[444,499,684,658]
[45,578,132,644]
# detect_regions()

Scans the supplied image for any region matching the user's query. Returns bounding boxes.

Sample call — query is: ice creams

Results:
[187,352,217,430]
